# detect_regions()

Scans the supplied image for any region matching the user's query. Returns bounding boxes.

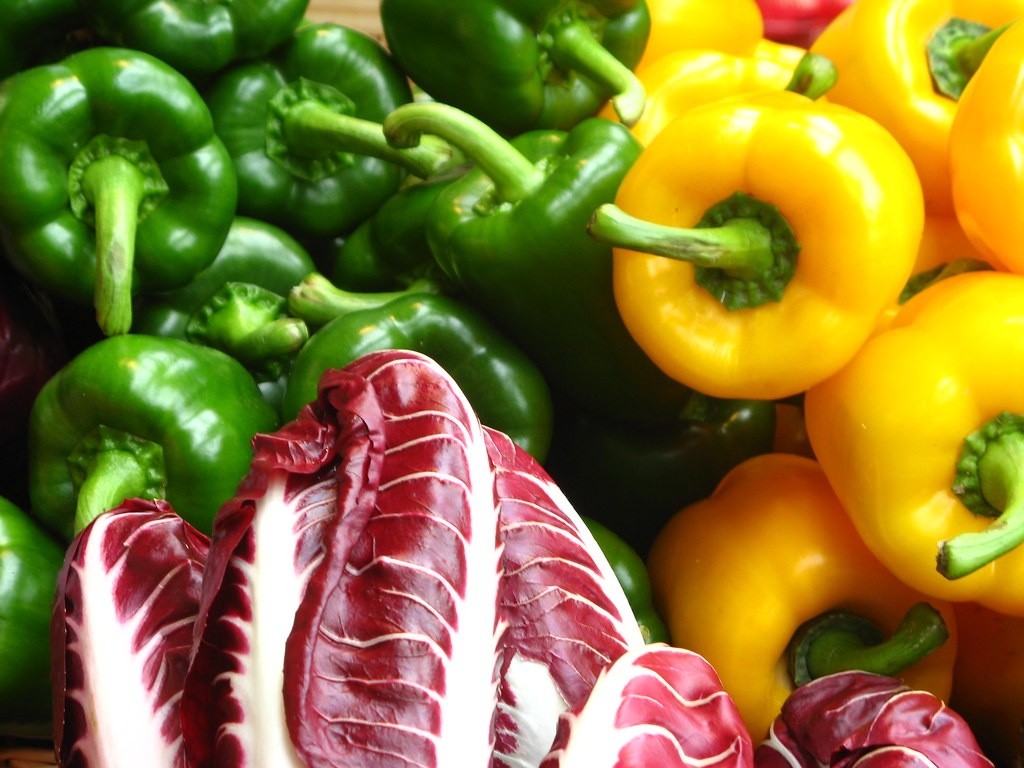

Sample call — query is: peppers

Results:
[597,0,1024,737]
[0,0,777,738]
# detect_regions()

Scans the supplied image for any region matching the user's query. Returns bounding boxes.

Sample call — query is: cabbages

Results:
[53,348,1002,766]
